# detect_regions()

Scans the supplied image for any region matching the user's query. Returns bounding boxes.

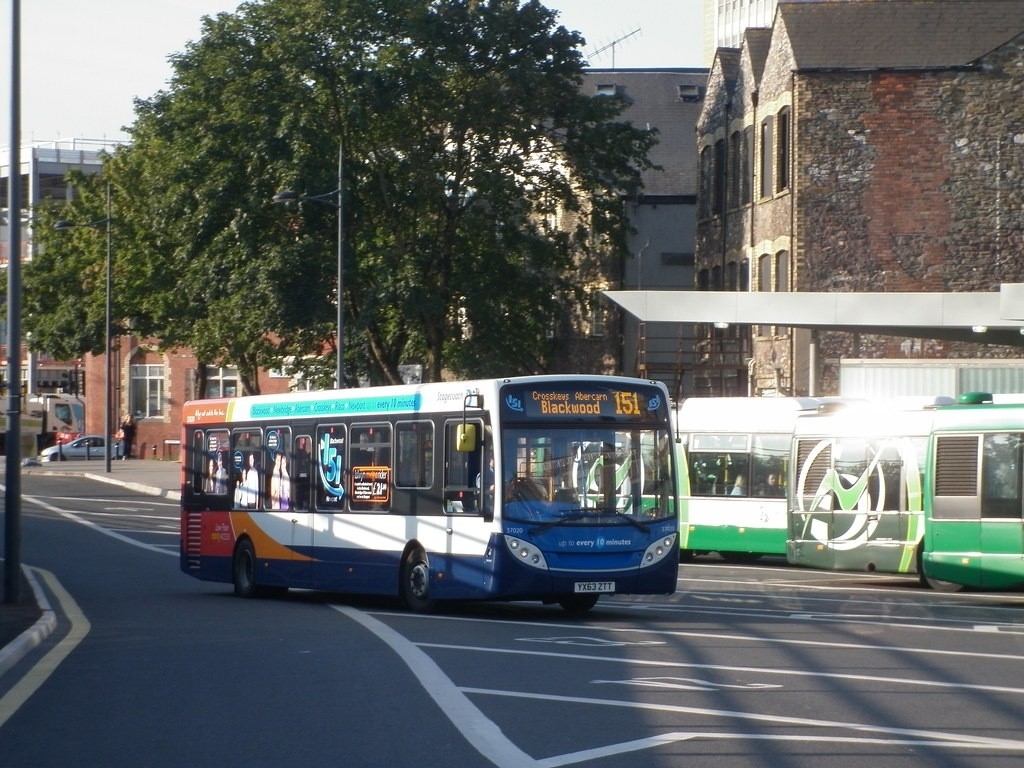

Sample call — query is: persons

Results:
[122,414,136,461]
[474,441,514,512]
[758,473,786,497]
[205,451,228,493]
[270,452,291,510]
[234,452,259,509]
[730,474,747,495]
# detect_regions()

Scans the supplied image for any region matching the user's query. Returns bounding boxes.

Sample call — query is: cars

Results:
[40,435,123,463]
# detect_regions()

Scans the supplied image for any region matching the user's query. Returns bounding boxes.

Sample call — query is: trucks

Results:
[0,391,85,455]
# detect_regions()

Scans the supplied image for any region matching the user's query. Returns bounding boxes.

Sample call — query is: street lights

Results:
[269,136,360,389]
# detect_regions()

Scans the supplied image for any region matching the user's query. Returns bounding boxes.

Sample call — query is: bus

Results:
[179,374,681,617]
[572,393,1024,595]
